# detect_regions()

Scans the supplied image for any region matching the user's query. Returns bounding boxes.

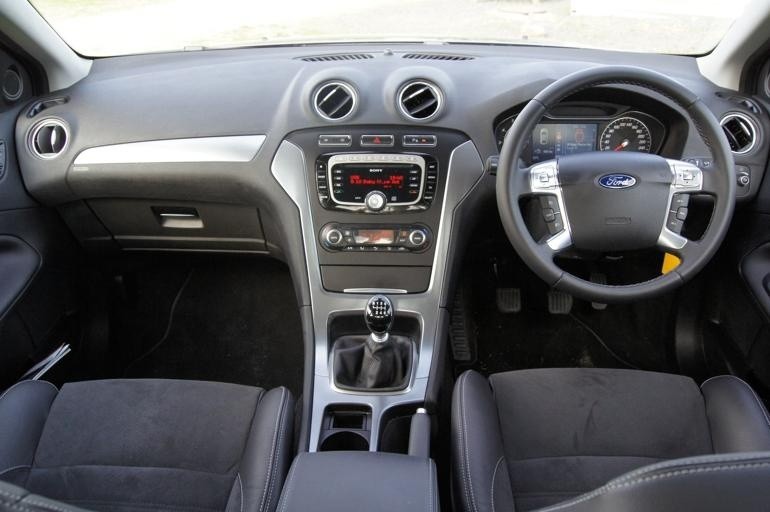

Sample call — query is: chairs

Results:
[1,378,290,511]
[453,368,769,509]
